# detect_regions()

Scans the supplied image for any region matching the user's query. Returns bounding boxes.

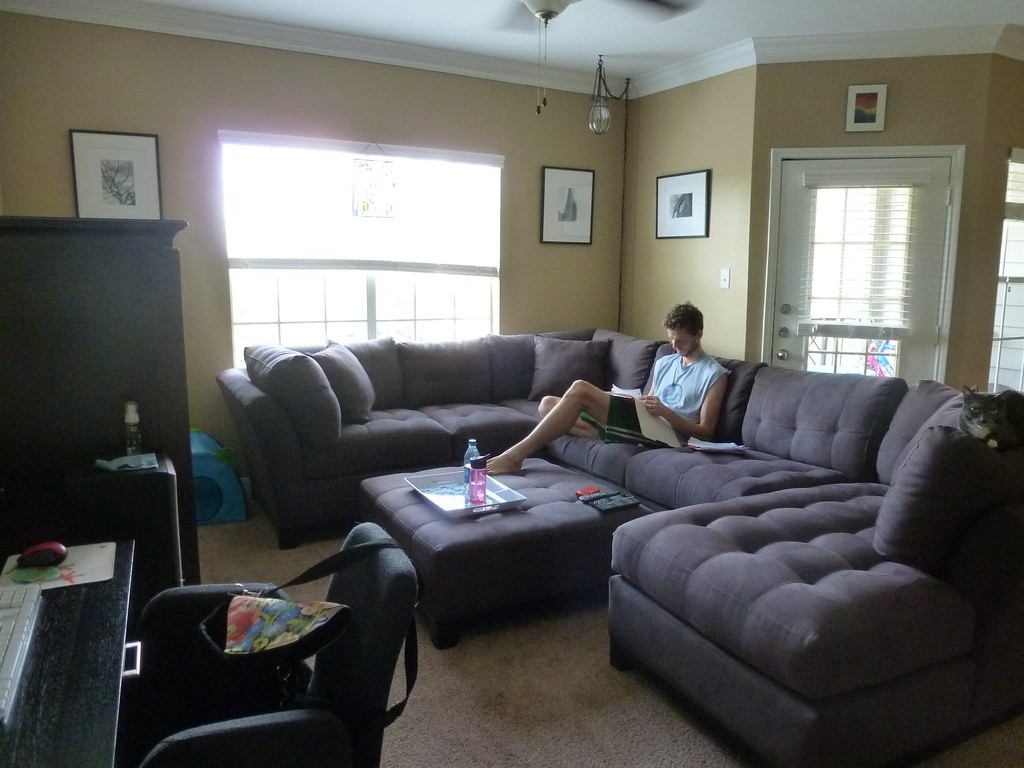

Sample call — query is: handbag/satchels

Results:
[196,597,292,722]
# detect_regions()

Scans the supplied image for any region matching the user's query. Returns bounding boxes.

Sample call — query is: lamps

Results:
[585,55,612,136]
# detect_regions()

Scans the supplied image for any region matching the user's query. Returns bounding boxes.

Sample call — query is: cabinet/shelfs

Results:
[0,213,202,639]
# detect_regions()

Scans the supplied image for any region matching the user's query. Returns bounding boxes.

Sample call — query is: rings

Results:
[649,405,651,408]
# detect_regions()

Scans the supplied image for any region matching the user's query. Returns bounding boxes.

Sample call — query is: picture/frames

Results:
[654,169,711,239]
[68,127,162,219]
[844,84,887,132]
[540,166,596,245]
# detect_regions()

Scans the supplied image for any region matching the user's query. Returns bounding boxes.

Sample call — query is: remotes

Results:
[578,488,641,512]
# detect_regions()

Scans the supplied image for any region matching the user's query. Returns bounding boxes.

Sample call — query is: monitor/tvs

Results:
[54,452,183,630]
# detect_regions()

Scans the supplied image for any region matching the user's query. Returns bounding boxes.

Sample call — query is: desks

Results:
[0,540,135,768]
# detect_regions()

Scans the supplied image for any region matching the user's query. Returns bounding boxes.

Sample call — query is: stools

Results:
[358,456,658,648]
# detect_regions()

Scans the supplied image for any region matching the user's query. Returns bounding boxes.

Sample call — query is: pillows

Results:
[310,340,377,422]
[527,333,615,395]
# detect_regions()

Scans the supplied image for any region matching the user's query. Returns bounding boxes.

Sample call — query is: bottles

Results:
[463,439,481,502]
[122,401,142,457]
[469,453,492,504]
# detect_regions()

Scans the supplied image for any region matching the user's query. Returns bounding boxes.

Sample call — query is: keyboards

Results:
[0,584,43,726]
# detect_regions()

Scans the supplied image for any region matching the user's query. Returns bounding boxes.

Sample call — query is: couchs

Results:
[216,321,1023,768]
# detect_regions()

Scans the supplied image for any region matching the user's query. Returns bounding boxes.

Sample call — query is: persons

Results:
[485,300,731,475]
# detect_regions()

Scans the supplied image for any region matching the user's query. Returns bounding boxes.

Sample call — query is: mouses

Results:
[16,542,68,567]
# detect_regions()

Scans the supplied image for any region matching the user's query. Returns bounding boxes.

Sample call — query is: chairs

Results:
[131,524,417,768]
